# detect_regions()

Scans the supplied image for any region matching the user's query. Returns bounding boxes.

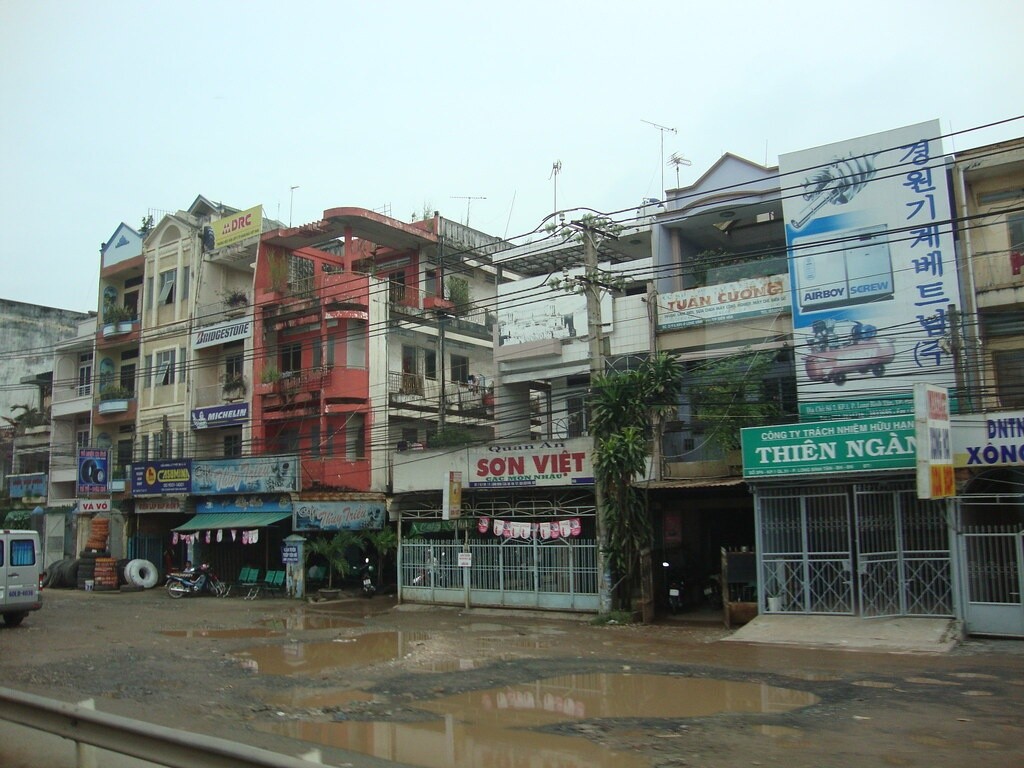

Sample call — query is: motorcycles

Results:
[353,558,376,599]
[164,562,223,599]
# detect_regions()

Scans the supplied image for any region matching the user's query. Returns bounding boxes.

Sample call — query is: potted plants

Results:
[482,380,494,404]
[309,529,367,601]
[214,284,249,317]
[101,298,132,338]
[98,383,131,415]
[219,372,246,400]
[255,366,280,396]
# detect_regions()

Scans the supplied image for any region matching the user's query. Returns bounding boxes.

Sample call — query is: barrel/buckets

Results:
[85,580,94,591]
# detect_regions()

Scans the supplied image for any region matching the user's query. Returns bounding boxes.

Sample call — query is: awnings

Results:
[171,512,293,531]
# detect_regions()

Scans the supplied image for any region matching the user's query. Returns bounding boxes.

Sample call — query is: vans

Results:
[0,528,43,625]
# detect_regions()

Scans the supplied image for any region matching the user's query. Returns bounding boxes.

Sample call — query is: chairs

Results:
[217,568,287,601]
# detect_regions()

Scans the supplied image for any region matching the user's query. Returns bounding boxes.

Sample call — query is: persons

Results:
[184,561,192,572]
[164,543,174,573]
